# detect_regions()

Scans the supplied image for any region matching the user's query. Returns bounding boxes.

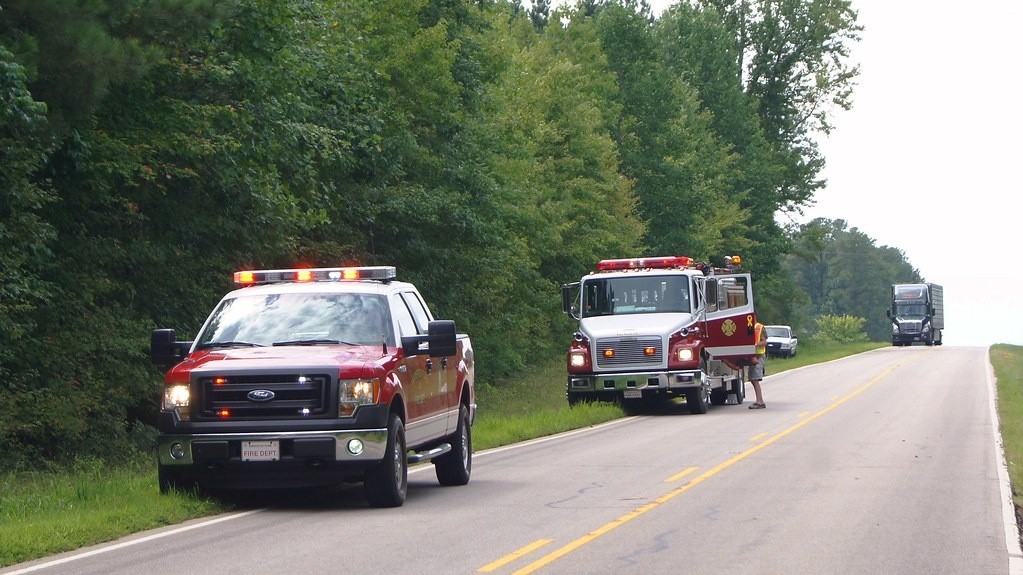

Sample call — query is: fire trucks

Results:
[559,256,758,414]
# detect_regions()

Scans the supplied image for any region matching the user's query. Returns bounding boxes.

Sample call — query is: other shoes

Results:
[748,403,766,409]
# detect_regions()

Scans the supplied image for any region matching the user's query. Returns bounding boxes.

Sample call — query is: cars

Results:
[764,326,797,359]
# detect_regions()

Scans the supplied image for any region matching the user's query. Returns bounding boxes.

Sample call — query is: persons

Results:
[748,310,768,409]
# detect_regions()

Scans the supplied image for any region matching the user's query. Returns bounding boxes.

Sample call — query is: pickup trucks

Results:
[149,266,477,509]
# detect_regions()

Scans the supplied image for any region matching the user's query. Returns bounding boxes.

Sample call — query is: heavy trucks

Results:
[886,283,944,346]
